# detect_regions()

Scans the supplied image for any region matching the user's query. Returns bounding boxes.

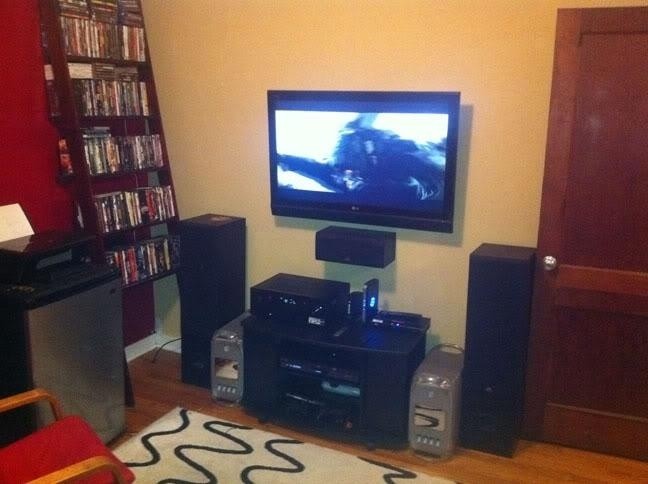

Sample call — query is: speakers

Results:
[317,227,396,269]
[406,343,465,459]
[178,215,245,387]
[459,244,535,458]
[210,312,250,402]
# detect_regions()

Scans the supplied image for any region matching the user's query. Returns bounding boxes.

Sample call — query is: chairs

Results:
[0,389,135,484]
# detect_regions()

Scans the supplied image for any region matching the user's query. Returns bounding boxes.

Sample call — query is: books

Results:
[77,184,175,233]
[105,237,180,283]
[45,1,152,117]
[60,127,164,177]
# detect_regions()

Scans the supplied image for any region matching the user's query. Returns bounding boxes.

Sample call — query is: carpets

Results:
[112,406,457,484]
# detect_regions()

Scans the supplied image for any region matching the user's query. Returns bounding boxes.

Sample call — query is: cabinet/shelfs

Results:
[40,0,179,407]
[241,311,430,451]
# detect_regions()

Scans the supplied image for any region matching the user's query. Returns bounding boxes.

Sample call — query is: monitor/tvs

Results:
[267,90,460,234]
